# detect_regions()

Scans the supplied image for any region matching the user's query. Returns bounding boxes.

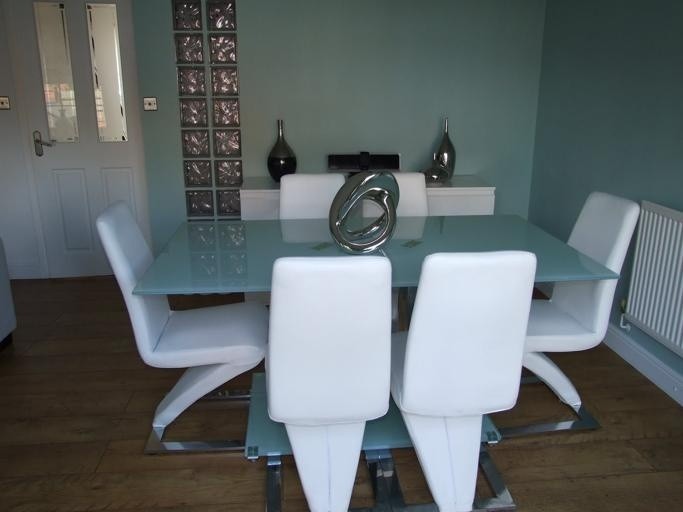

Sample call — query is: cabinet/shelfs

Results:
[237,176,496,308]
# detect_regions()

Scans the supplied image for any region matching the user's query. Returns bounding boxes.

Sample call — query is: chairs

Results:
[379,249,536,511]
[361,170,429,216]
[278,171,347,220]
[240,255,393,510]
[93,199,268,456]
[496,190,641,441]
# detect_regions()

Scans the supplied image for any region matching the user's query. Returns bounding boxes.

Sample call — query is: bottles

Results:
[431,118,455,179]
[419,151,448,187]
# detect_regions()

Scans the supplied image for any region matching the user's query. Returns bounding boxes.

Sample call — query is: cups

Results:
[359,152,369,170]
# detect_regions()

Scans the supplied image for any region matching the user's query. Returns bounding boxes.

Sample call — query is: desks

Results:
[129,219,619,511]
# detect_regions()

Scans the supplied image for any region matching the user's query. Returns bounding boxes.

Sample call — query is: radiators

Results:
[619,199,682,357]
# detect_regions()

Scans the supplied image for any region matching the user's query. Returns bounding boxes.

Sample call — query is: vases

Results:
[435,115,456,179]
